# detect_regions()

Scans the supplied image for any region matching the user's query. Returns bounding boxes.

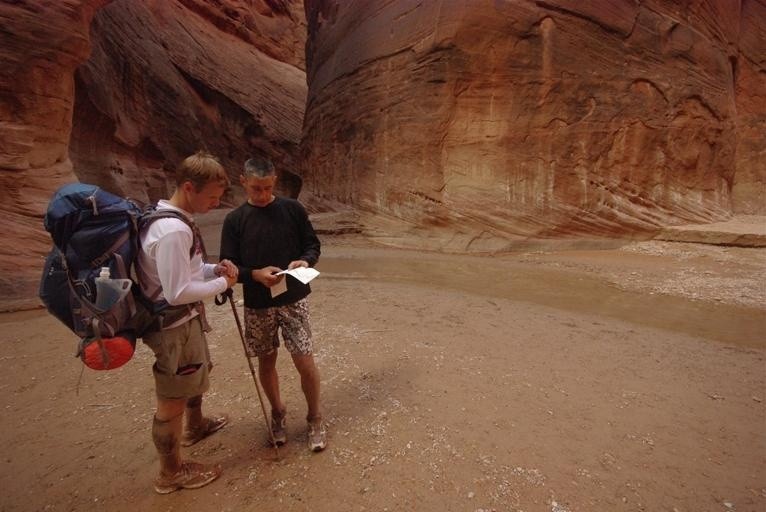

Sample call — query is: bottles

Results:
[96,266,114,311]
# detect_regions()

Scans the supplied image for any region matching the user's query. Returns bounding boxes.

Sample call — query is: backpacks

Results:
[34,180,201,371]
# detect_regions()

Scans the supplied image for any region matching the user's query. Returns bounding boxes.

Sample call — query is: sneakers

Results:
[153,459,222,495]
[306,409,328,453]
[181,415,228,446]
[268,406,290,446]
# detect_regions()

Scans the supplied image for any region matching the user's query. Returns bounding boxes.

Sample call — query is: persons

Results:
[220,157,329,452]
[134,150,240,494]
